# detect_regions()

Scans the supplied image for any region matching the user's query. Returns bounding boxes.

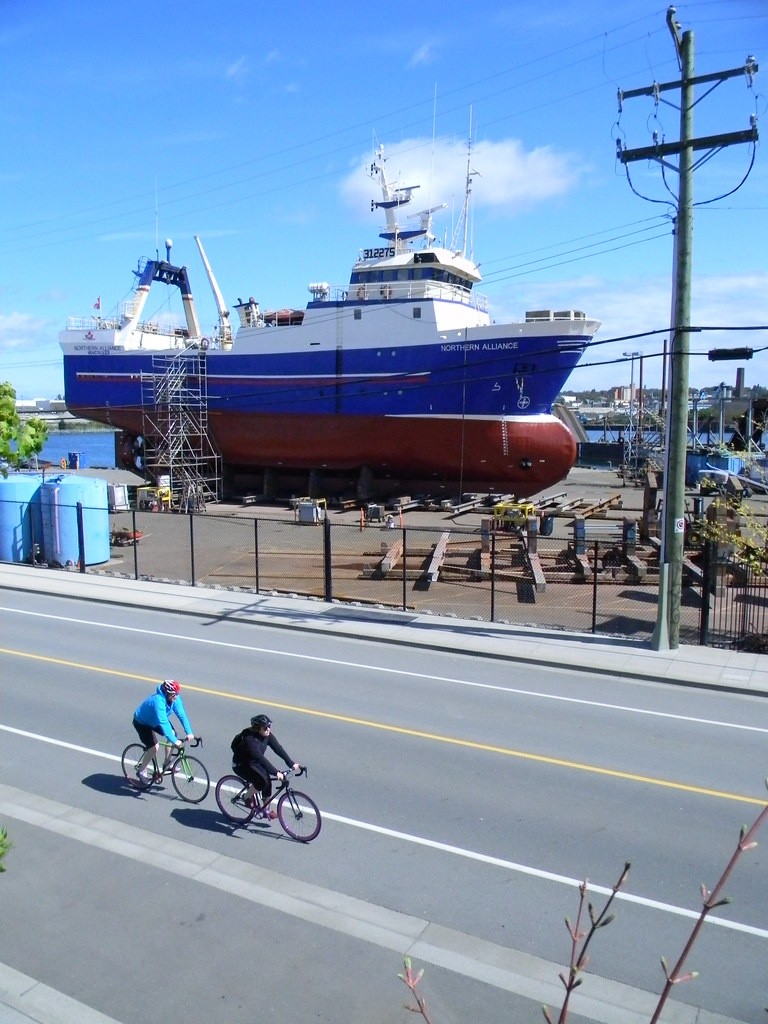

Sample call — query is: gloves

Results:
[186,734,194,741]
[174,740,183,746]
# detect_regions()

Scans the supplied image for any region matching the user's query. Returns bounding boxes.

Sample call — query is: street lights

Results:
[623,351,643,463]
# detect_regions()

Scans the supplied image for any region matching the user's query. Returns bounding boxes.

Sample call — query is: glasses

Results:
[264,724,272,731]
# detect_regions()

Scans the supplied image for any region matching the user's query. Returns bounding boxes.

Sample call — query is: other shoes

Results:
[263,810,278,818]
[242,793,255,808]
[136,769,150,785]
[162,764,180,772]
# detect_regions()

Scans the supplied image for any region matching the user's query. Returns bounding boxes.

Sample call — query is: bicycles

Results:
[214,765,322,843]
[121,737,211,804]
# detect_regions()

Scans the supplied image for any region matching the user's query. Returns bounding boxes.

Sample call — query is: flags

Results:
[91,297,100,309]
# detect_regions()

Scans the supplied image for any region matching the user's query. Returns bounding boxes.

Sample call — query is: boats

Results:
[263,307,305,323]
[57,80,600,502]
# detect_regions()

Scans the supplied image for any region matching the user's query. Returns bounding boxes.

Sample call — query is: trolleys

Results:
[116,529,152,547]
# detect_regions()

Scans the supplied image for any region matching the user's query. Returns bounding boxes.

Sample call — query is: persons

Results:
[231,715,300,818]
[133,680,194,785]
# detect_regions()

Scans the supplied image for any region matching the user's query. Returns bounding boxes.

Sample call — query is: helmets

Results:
[162,680,181,693]
[251,714,272,729]
